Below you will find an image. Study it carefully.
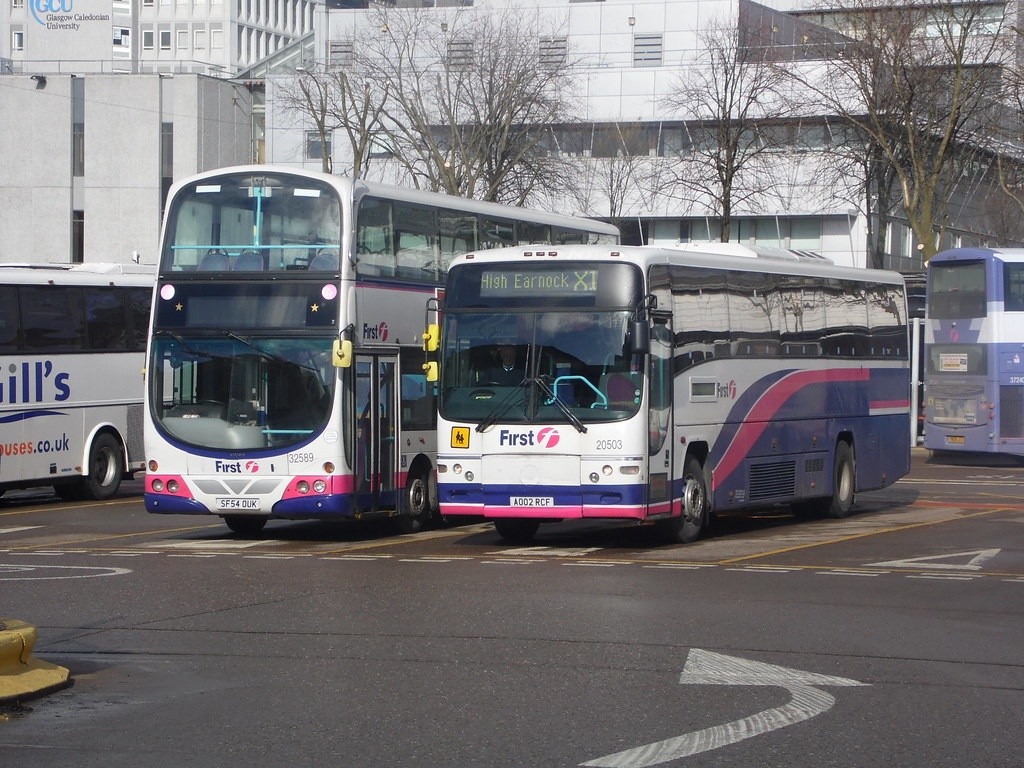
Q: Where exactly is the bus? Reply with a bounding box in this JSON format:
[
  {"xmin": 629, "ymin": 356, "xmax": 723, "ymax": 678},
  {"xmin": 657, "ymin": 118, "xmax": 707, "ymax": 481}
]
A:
[
  {"xmin": 922, "ymin": 248, "xmax": 1024, "ymax": 457},
  {"xmin": 140, "ymin": 163, "xmax": 620, "ymax": 536},
  {"xmin": 419, "ymin": 241, "xmax": 914, "ymax": 543},
  {"xmin": 0, "ymin": 262, "xmax": 164, "ymax": 501}
]
